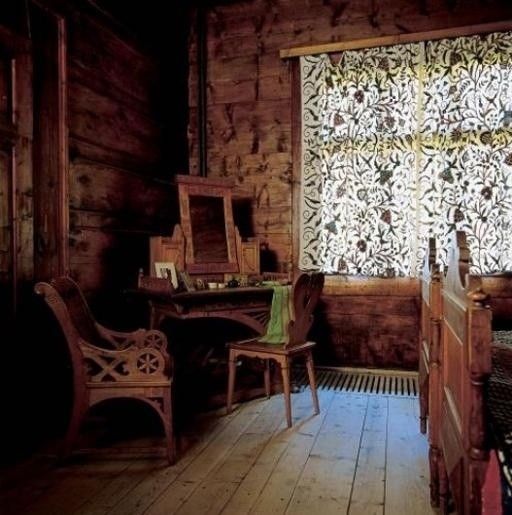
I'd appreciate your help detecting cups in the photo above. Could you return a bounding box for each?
[207,282,225,289]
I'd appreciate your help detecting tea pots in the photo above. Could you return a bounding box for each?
[227,277,241,288]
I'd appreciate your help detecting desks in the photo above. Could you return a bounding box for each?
[140,277,291,401]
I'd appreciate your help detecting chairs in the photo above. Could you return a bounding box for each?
[224,271,327,429]
[36,276,179,468]
